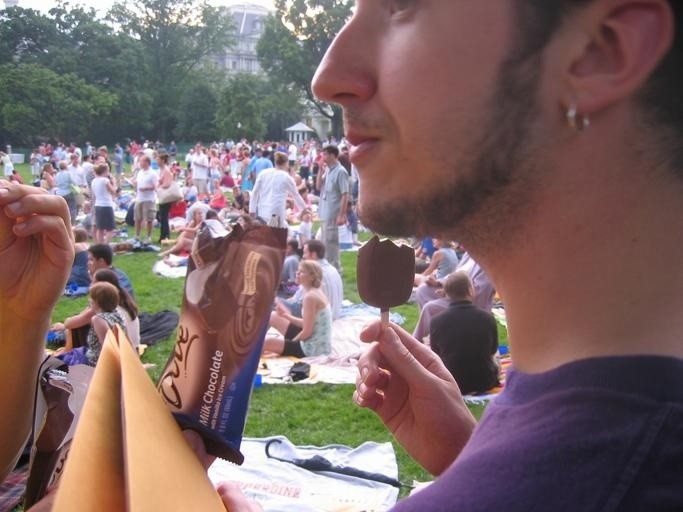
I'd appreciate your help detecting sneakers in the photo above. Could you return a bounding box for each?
[131,235,138,241]
[143,236,151,244]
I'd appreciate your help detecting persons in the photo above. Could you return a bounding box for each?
[82,141,112,172]
[412,252,496,346]
[184,138,250,211]
[218,0,682,512]
[159,202,269,268]
[86,155,114,243]
[261,259,332,358]
[64,269,140,357]
[297,209,312,257]
[98,145,111,172]
[67,229,92,287]
[316,145,349,278]
[87,244,134,302]
[39,154,82,225]
[247,135,288,180]
[287,142,297,173]
[271,240,343,323]
[280,240,299,281]
[249,152,306,230]
[10,170,23,184]
[0,182,218,512]
[55,283,134,367]
[430,273,500,396]
[126,269,127,270]
[31,143,81,178]
[287,167,317,212]
[298,140,322,189]
[0,151,14,181]
[134,155,156,245]
[128,160,182,178]
[416,236,436,259]
[126,138,177,161]
[321,135,354,177]
[114,143,123,174]
[71,204,92,232]
[91,164,116,245]
[154,154,173,246]
[414,237,459,286]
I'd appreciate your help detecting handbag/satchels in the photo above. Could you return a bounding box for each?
[70,184,81,195]
[154,182,184,204]
